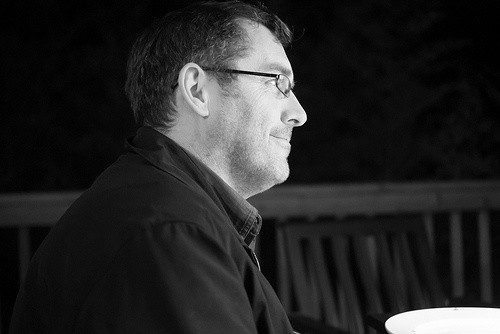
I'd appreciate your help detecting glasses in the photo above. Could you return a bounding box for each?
[171,66,295,98]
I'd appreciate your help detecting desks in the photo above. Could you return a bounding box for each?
[384,307,500,334]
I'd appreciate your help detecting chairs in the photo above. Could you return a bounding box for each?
[277,220,438,334]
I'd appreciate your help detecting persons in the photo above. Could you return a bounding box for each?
[9,0,308,334]
